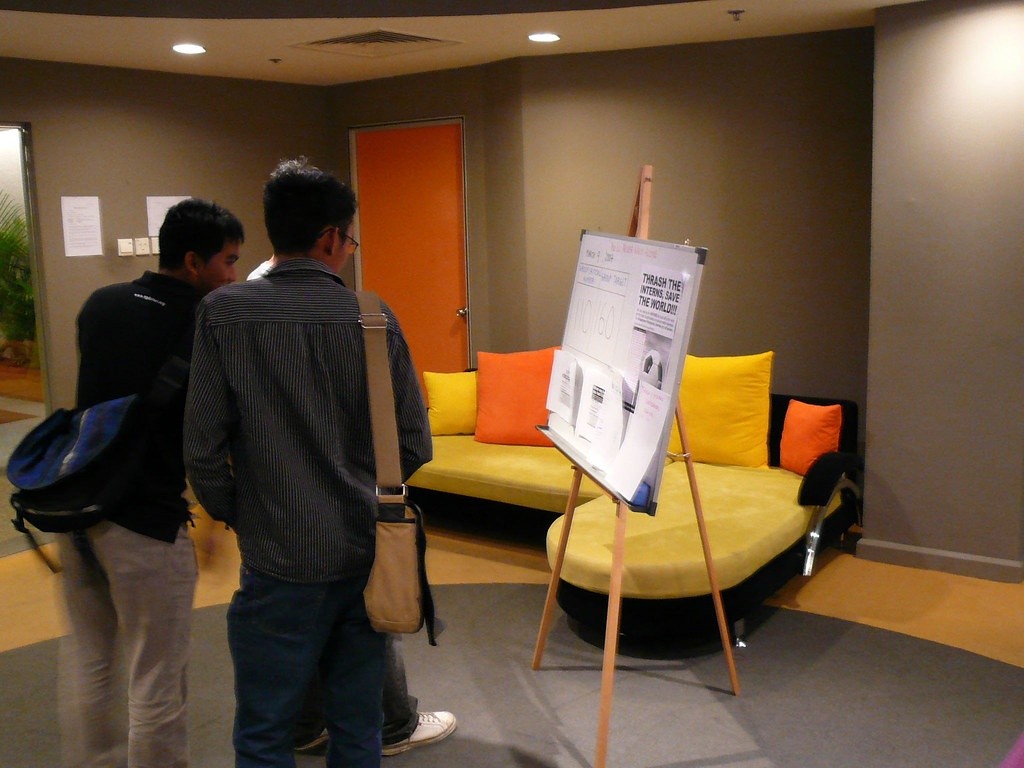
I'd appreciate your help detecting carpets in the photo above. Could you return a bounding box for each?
[0,581,1024,768]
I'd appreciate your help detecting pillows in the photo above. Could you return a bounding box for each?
[779,399,843,476]
[422,370,479,434]
[474,345,563,448]
[665,352,774,469]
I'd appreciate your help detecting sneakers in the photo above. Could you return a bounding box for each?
[381,711,456,757]
[293,729,329,752]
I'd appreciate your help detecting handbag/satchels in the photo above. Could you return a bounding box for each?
[5,391,148,532]
[363,486,436,647]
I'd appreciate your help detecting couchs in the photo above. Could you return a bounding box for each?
[406,367,863,652]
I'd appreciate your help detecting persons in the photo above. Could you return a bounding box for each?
[55,197,245,768]
[183,162,433,768]
[247,253,457,756]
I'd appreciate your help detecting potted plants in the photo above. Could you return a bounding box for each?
[0,189,39,366]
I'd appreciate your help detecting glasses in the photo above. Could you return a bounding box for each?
[319,228,360,255]
